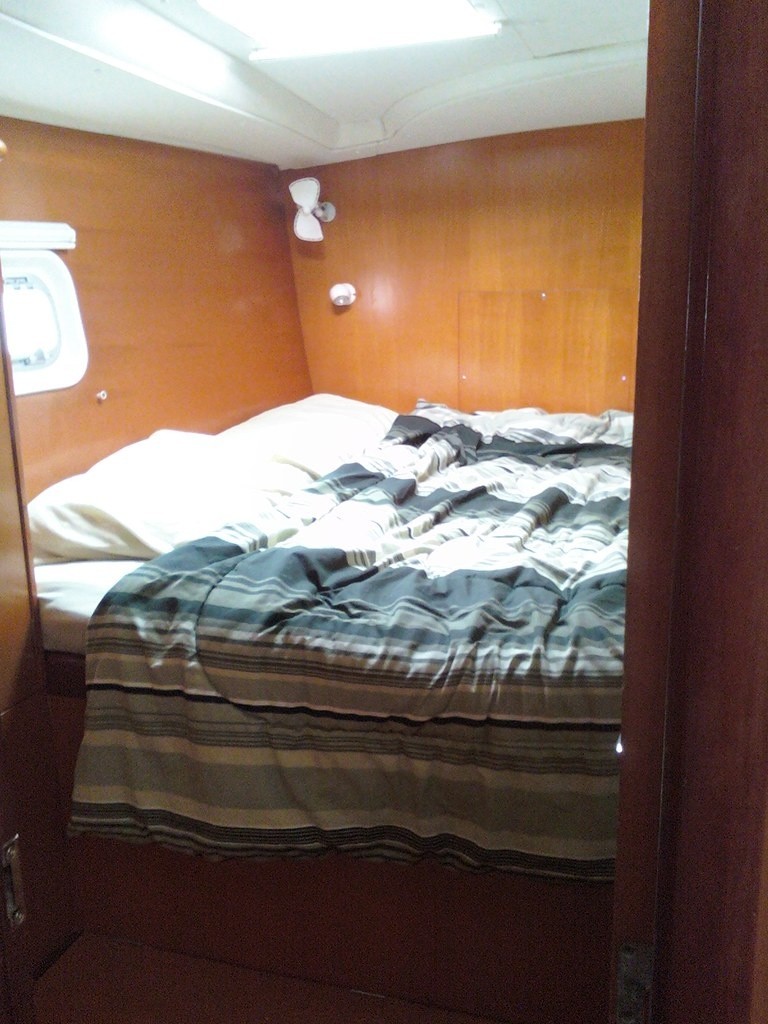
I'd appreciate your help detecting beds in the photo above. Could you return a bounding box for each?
[20,390,634,887]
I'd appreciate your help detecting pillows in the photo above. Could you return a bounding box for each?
[27,428,316,559]
[215,390,402,480]
